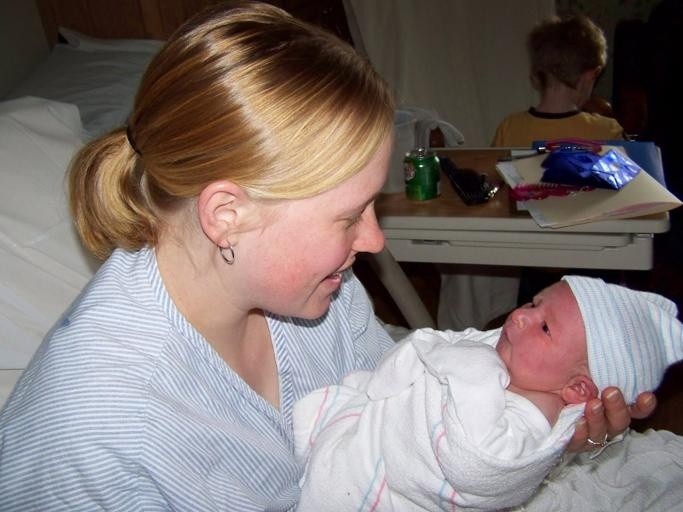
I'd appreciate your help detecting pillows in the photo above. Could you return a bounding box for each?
[5,28,166,140]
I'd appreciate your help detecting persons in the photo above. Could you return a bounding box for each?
[2,1,659,512]
[287,275,681,512]
[490,14,622,145]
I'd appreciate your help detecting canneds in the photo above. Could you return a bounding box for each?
[403,144,441,201]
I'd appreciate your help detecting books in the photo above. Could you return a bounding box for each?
[491,141,682,231]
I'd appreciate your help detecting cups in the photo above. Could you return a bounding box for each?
[378,107,419,196]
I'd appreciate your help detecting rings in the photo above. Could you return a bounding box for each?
[584,434,608,444]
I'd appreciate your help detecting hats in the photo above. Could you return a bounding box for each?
[561,274,683,407]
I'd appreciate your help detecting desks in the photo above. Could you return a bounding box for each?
[524,270,525,277]
[371,147,671,277]
